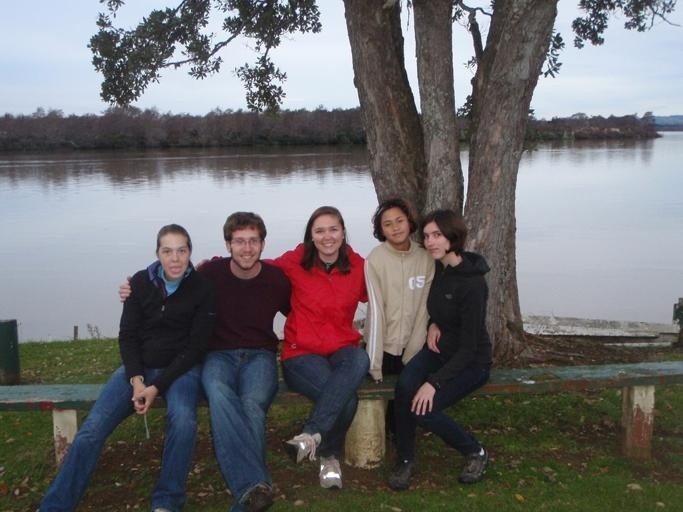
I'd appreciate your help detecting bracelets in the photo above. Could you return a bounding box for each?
[129,374,143,387]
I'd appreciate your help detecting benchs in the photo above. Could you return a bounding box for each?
[0,358,683,471]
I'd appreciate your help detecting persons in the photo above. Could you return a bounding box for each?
[30,222,216,512]
[361,197,435,445]
[386,209,492,493]
[115,210,292,512]
[192,206,368,490]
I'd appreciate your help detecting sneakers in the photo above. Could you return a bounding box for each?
[281,432,322,465]
[458,445,489,483]
[387,457,418,491]
[319,454,343,490]
[240,483,274,512]
